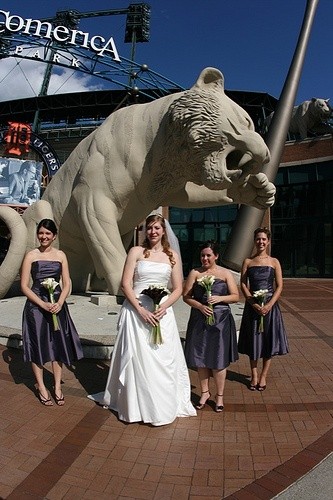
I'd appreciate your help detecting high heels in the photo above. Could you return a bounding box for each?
[37,387,53,406]
[215,393,224,412]
[54,388,65,406]
[196,391,211,410]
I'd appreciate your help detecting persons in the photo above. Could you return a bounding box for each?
[20,219,84,406]
[238,228,290,391]
[86,213,198,426]
[10,161,35,203]
[183,240,239,411]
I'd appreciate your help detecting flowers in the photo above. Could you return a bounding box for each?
[40,278,61,331]
[197,275,216,326]
[249,289,268,333]
[140,285,171,345]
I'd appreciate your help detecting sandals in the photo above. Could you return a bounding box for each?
[258,384,266,391]
[249,384,257,390]
